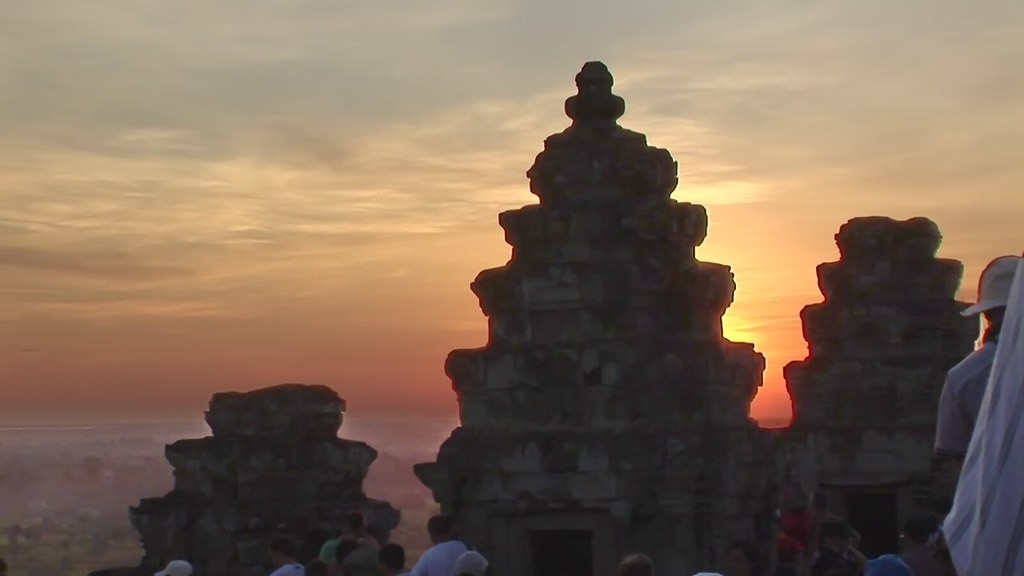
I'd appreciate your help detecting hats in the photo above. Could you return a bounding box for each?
[317,537,344,566]
[451,551,491,576]
[153,560,195,576]
[862,553,915,576]
[958,255,1022,320]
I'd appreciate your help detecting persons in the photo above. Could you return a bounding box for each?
[934,256,1024,576]
[620,484,952,576]
[140,508,487,576]
